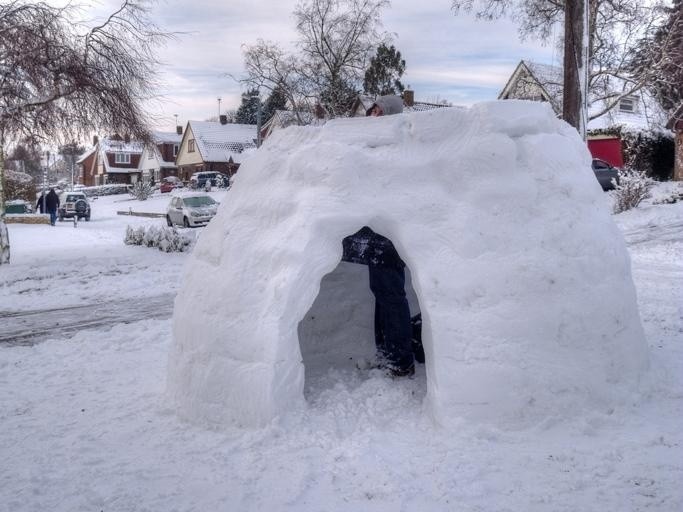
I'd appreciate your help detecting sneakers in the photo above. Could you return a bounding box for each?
[380,348,416,379]
[356,347,393,370]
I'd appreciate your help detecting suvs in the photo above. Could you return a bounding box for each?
[55,191,92,221]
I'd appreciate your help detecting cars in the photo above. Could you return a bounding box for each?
[166,192,221,229]
[159,175,184,194]
[591,157,628,194]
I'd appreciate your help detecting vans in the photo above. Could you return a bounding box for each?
[189,170,230,188]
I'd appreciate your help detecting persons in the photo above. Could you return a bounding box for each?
[46,189,60,225]
[36,192,47,213]
[341,96,425,378]
